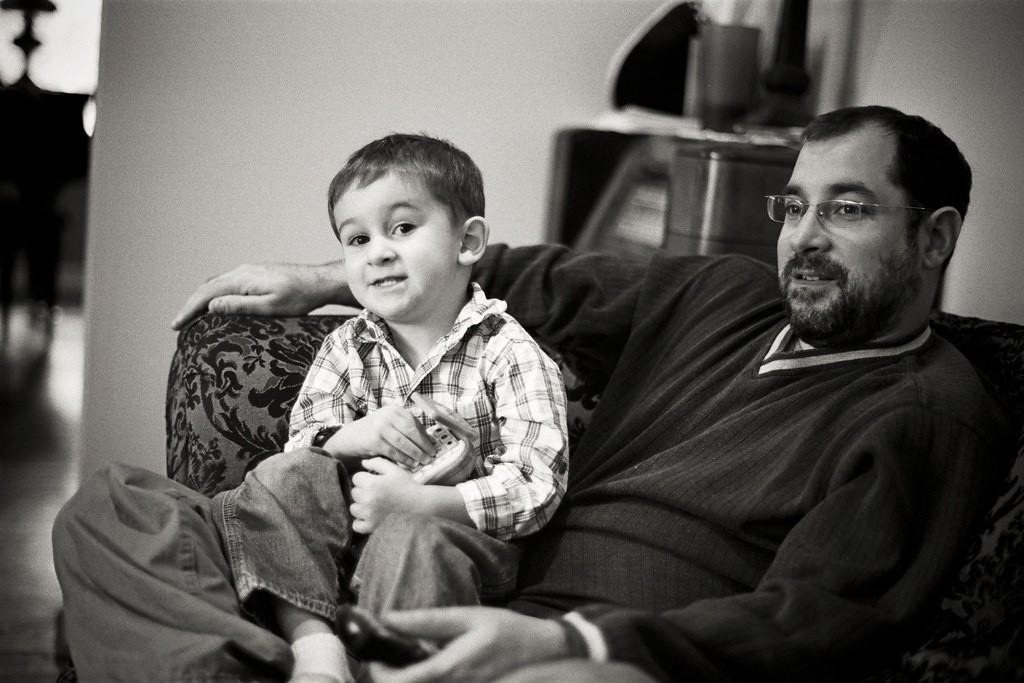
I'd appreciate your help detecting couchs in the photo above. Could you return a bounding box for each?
[169,309,1024,683]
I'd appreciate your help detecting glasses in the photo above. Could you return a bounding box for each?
[764,193,931,229]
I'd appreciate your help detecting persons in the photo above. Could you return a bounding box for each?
[50,105,1001,683]
[209,132,573,683]
